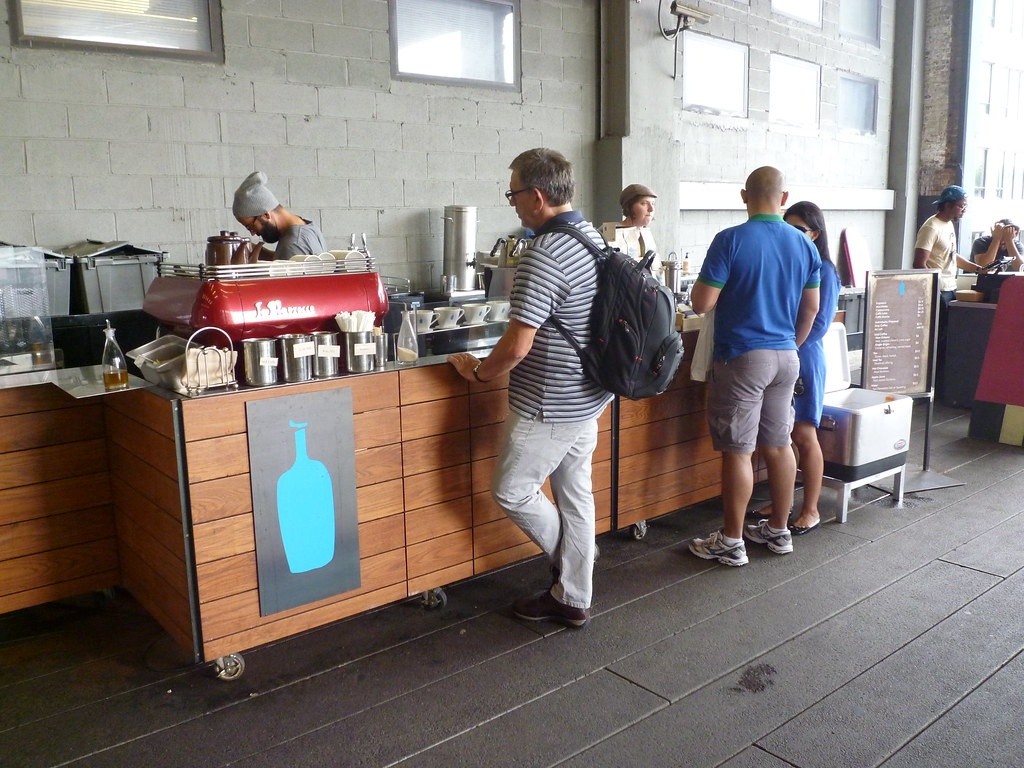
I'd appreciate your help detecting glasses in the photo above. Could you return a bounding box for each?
[954,204,968,212]
[504,187,532,202]
[245,216,257,230]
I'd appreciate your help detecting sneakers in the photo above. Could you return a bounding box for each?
[688,527,749,566]
[744,519,794,554]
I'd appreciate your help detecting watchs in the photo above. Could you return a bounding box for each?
[472,363,491,384]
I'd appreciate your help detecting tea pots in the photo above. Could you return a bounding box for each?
[491,235,533,268]
[205,230,264,265]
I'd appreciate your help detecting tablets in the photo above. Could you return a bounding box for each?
[975,256,1017,274]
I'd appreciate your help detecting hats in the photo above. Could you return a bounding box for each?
[619,183,658,208]
[232,170,280,220]
[930,185,968,209]
[995,220,1019,238]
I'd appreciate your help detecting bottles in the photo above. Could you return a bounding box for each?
[682,253,690,272]
[102,321,129,391]
[28,308,51,365]
[396,310,419,366]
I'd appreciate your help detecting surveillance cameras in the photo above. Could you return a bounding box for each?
[671,0,713,25]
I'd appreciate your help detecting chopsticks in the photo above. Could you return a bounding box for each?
[335,309,376,332]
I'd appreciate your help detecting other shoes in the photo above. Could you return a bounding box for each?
[747,509,794,520]
[547,543,600,589]
[513,589,591,629]
[789,517,821,535]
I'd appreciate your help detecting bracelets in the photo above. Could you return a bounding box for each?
[975,265,981,275]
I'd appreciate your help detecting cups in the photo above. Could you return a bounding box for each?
[434,307,465,326]
[410,309,440,330]
[461,303,491,322]
[240,330,389,386]
[486,301,511,319]
[262,249,366,276]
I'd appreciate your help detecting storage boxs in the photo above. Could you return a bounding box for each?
[0,244,75,317]
[955,290,984,302]
[125,335,202,391]
[54,238,171,314]
[798,322,915,482]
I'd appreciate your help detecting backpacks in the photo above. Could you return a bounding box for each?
[529,224,684,400]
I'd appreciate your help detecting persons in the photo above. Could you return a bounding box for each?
[913,185,990,395]
[231,172,327,261]
[747,201,840,538]
[971,218,1024,303]
[619,184,665,289]
[448,149,613,625]
[689,165,820,568]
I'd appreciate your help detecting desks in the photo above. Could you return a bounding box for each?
[940,299,997,426]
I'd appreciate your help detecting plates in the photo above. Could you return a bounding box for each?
[419,318,510,332]
